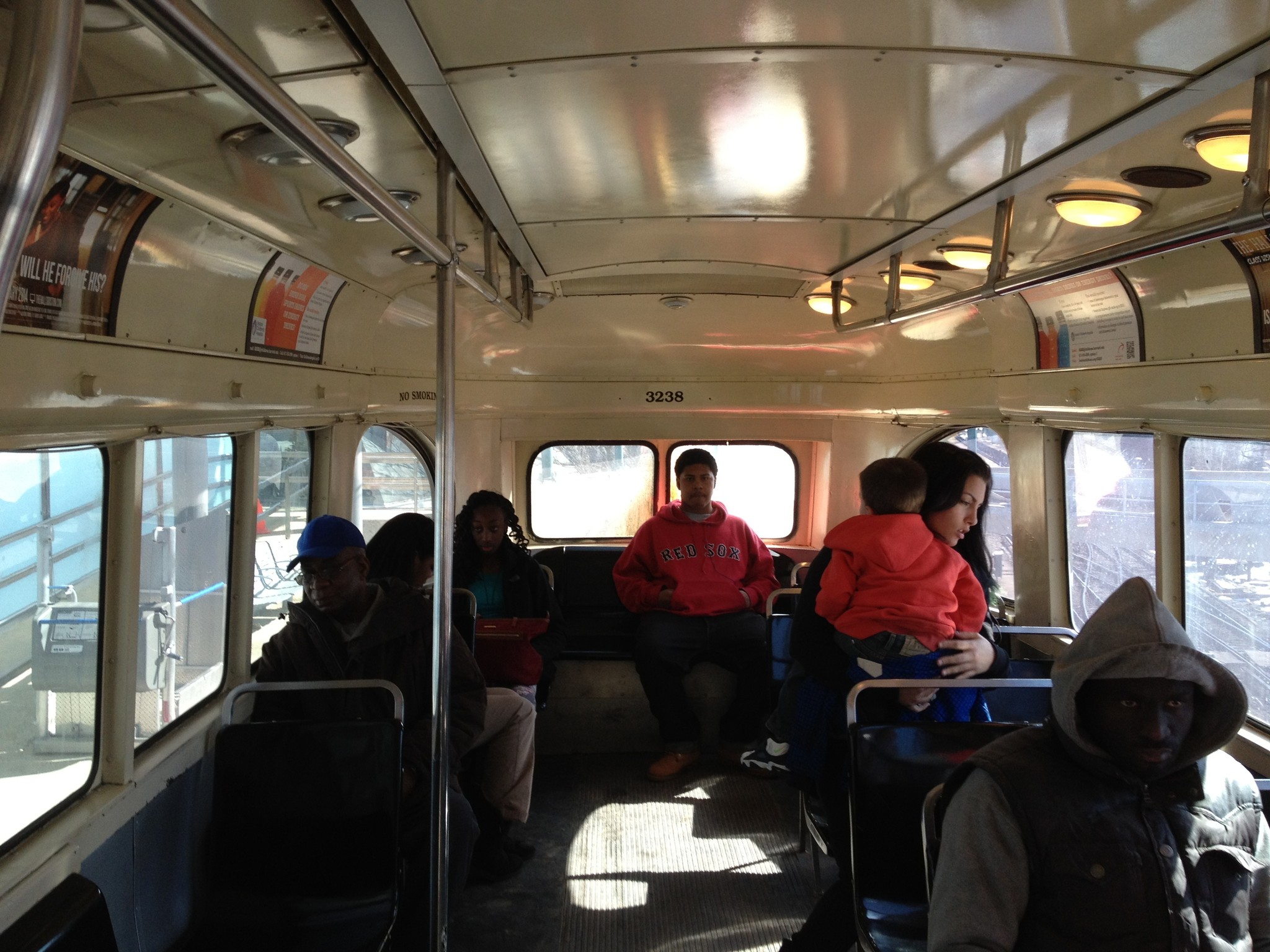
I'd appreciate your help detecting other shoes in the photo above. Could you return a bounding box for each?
[468,844,524,885]
[482,827,534,862]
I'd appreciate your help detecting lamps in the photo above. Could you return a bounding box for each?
[1181,125,1254,173]
[80,0,144,35]
[219,116,361,166]
[936,245,1016,270]
[392,242,469,266]
[659,295,693,310]
[504,292,555,313]
[878,270,940,291]
[318,190,421,224]
[1045,192,1155,229]
[803,293,856,315]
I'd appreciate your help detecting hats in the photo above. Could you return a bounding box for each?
[286,514,366,572]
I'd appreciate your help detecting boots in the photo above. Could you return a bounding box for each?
[645,741,700,782]
[719,741,773,777]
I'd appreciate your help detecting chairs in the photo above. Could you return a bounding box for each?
[173,679,409,952]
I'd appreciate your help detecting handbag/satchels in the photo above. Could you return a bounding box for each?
[473,613,550,685]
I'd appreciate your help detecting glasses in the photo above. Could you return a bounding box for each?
[294,555,360,586]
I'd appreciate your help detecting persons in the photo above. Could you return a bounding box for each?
[19,174,79,328]
[360,512,536,864]
[611,446,781,781]
[924,575,1270,952]
[775,440,1013,952]
[451,491,557,731]
[254,514,491,940]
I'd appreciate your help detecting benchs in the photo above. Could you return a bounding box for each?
[533,547,800,663]
[767,561,1270,952]
